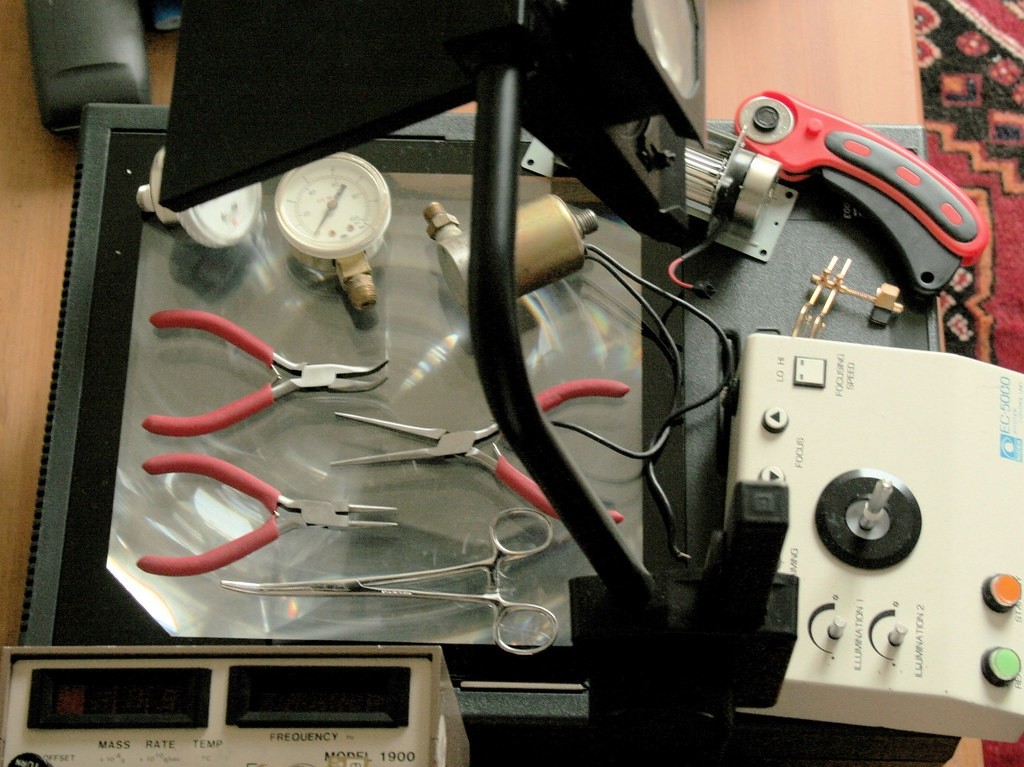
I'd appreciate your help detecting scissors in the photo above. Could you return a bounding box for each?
[220,508,557,654]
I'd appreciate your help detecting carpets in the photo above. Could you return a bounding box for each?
[910,0,1024,767]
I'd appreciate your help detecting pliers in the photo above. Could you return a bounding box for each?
[142,308,390,438]
[137,454,399,577]
[330,378,630,524]
